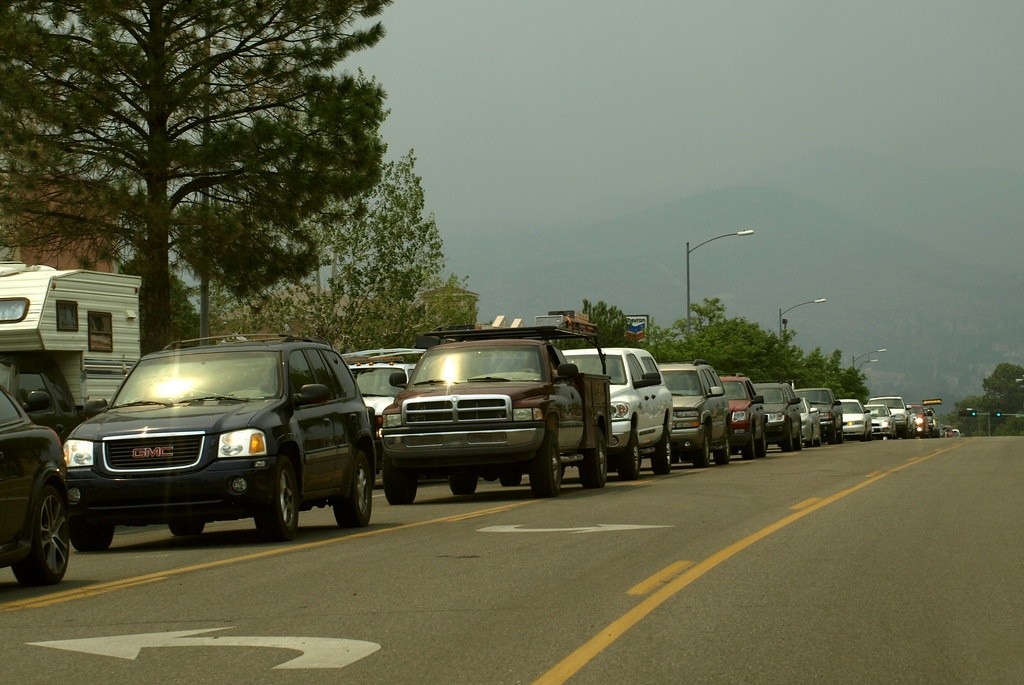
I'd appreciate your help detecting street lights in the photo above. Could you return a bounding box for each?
[852,348,887,369]
[687,229,754,336]
[782,318,788,330]
[779,297,827,340]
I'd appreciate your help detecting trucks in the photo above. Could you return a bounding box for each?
[0,259,143,445]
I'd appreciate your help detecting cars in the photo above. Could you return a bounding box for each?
[800,399,823,447]
[925,407,960,438]
[910,404,930,436]
[909,410,917,437]
[836,399,872,441]
[1,383,69,590]
[864,405,896,439]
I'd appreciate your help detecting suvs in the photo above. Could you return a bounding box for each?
[61,332,373,549]
[751,382,803,452]
[559,350,674,477]
[716,372,769,460]
[794,388,844,446]
[868,397,913,438]
[660,360,732,468]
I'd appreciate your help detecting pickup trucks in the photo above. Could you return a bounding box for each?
[379,308,613,506]
[343,348,427,471]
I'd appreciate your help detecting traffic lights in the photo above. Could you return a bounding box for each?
[991,412,1001,417]
[959,410,977,416]
